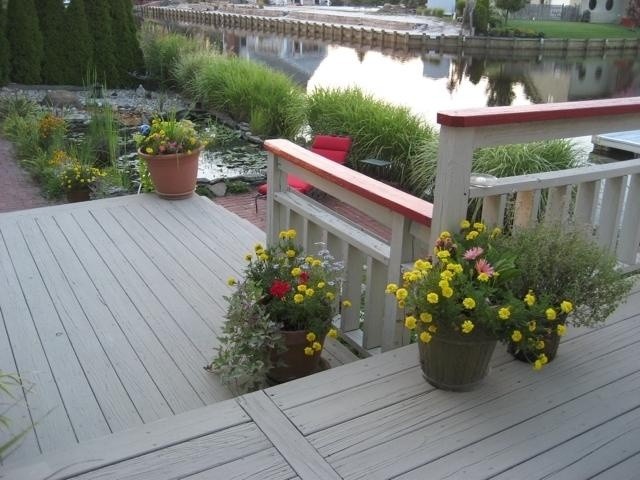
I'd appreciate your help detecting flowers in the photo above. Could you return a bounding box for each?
[56,164,110,192]
[130,82,217,170]
[382,219,573,370]
[202,227,352,399]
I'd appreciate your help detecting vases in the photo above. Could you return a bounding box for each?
[66,184,92,202]
[138,145,206,200]
[415,315,499,393]
[262,318,331,387]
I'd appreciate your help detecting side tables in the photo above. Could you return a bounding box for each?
[357,157,395,187]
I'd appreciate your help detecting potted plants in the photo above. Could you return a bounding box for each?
[497,220,640,366]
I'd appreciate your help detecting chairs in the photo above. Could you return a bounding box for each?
[254,133,353,214]
